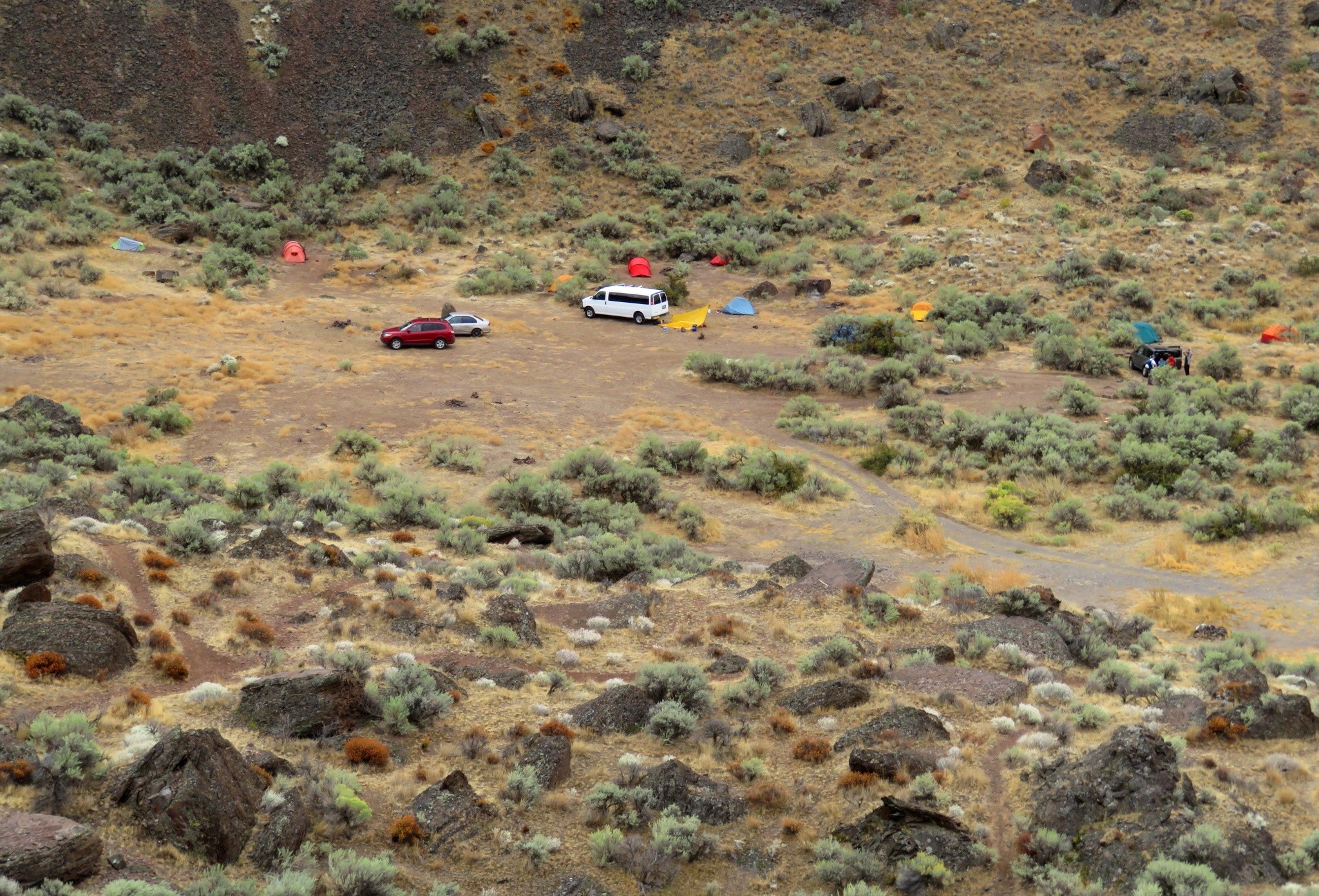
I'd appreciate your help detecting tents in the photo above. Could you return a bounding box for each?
[1262,325,1299,344]
[1130,322,1162,344]
[628,257,654,278]
[549,273,573,294]
[909,302,934,321]
[721,297,757,316]
[283,241,307,264]
[710,254,733,265]
[110,237,147,252]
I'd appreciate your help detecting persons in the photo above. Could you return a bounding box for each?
[1166,354,1177,369]
[1158,358,1167,367]
[1183,348,1193,376]
[1148,355,1158,385]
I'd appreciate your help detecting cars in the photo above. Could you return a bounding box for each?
[439,313,491,339]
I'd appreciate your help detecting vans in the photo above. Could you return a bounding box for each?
[580,283,669,325]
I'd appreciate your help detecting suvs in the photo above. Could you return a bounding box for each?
[381,318,456,350]
[1129,344,1183,377]
